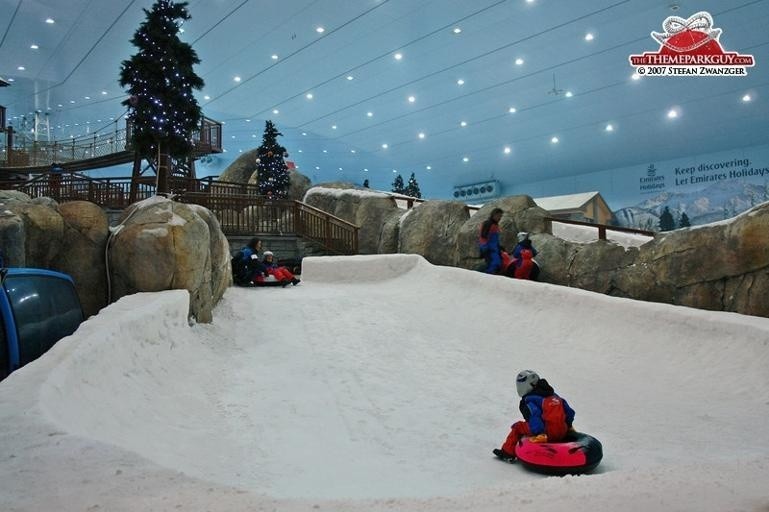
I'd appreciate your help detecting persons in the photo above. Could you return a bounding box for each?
[232,237,269,287]
[492,368,576,463]
[478,207,512,274]
[259,249,302,288]
[506,230,541,281]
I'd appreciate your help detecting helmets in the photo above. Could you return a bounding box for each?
[515,369,540,396]
[263,250,274,259]
[516,231,529,243]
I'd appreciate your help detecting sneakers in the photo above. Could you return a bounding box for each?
[281,279,302,288]
[492,447,516,463]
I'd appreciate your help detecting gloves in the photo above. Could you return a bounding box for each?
[528,434,548,443]
[570,426,577,433]
